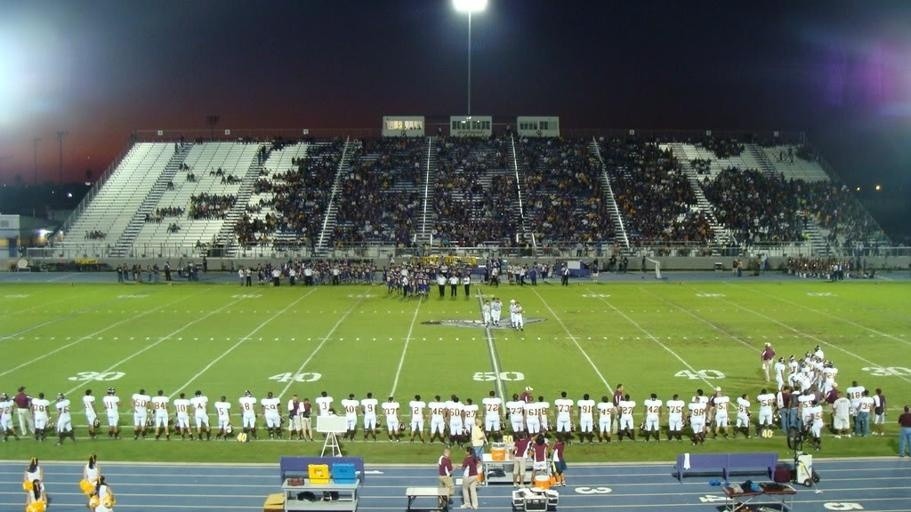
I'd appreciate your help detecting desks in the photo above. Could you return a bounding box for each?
[723,484,797,512]
[282,477,359,512]
[405,486,452,512]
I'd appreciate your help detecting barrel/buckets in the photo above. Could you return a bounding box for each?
[535,475,550,489]
[491,446,506,462]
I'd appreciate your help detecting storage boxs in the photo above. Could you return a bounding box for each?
[263,493,285,512]
[309,464,358,483]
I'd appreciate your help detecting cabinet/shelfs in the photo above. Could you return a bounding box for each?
[483,453,549,486]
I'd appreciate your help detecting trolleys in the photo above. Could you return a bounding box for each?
[792,431,820,487]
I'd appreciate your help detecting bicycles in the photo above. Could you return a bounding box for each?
[788,412,821,450]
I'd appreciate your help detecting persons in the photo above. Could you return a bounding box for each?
[82,454,114,512]
[435,418,567,509]
[0,381,886,444]
[1,131,911,299]
[482,297,523,331]
[897,405,911,458]
[761,342,839,403]
[23,457,48,512]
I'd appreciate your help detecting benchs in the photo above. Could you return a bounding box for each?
[281,457,365,485]
[671,451,778,481]
[48,143,892,261]
